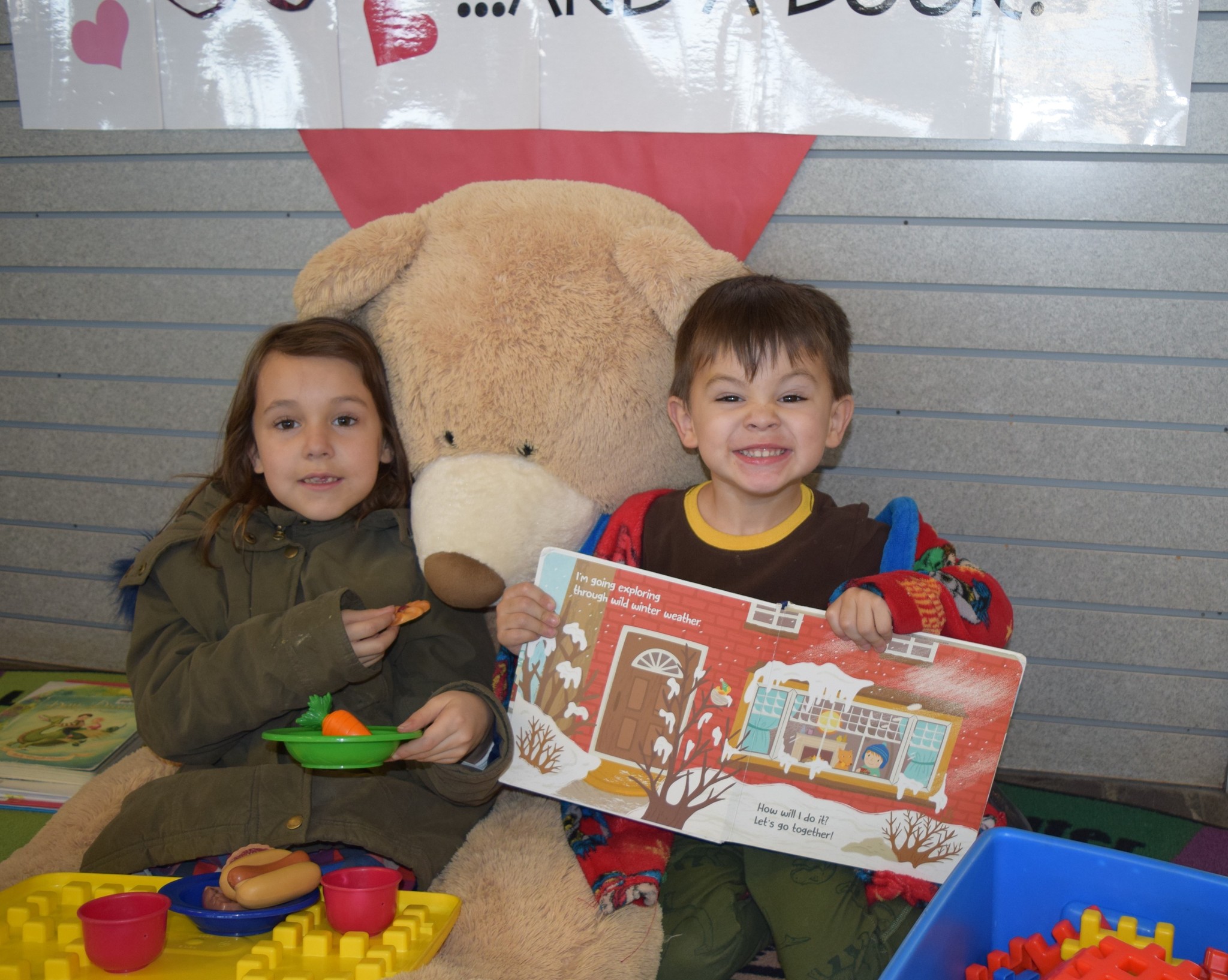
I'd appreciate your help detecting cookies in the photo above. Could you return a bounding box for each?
[390,600,431,625]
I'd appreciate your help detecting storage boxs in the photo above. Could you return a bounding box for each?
[879,826,1228,980]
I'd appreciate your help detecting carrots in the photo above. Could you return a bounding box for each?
[296,692,372,736]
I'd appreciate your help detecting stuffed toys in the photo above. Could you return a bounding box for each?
[0,177,747,979]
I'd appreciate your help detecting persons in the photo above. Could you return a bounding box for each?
[83,318,514,892]
[494,276,1013,979]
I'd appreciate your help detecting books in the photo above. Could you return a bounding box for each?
[0,678,144,812]
[497,547,1028,886]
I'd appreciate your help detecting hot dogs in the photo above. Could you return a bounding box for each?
[219,849,322,909]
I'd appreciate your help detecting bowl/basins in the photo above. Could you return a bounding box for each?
[77,891,171,973]
[321,866,403,937]
[157,872,321,937]
[262,725,423,769]
